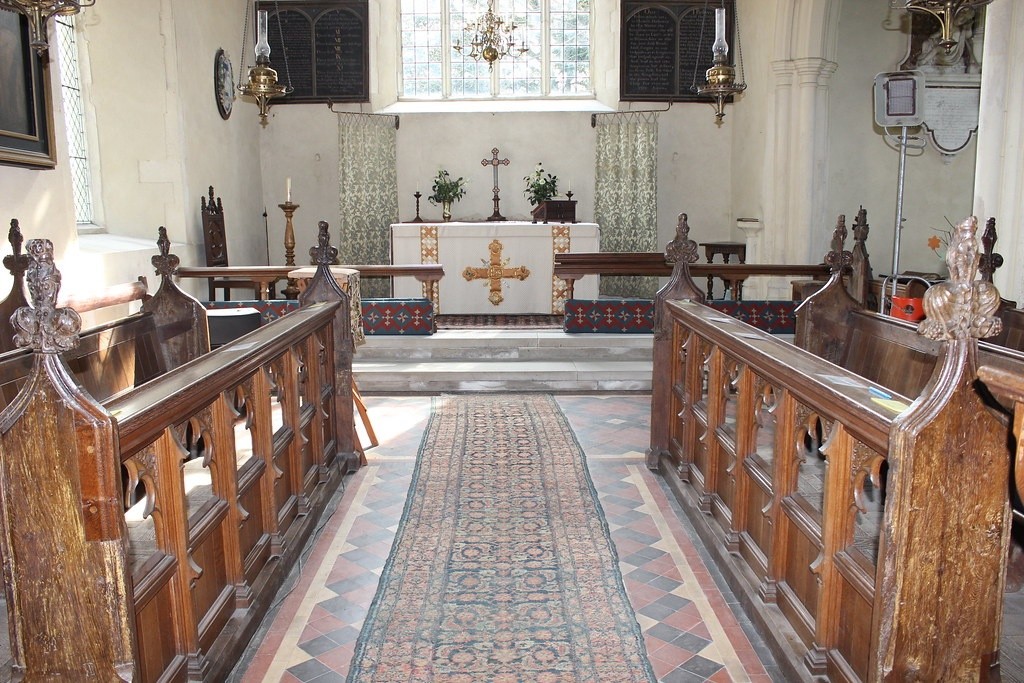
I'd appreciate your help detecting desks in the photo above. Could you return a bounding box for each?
[699,241,746,300]
[389,221,601,314]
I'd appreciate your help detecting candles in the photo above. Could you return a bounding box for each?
[287,178,291,201]
[569,180,570,191]
[417,183,418,192]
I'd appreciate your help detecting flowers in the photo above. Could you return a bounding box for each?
[523,163,563,206]
[428,170,471,206]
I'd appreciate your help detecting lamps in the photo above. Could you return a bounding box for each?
[453,0,529,72]
[237,0,294,121]
[690,0,747,120]
[888,0,994,55]
[0,0,95,57]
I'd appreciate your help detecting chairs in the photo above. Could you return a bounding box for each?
[201,185,280,301]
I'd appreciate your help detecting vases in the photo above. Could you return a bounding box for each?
[443,200,451,222]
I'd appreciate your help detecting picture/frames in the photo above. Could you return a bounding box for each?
[0,10,59,169]
